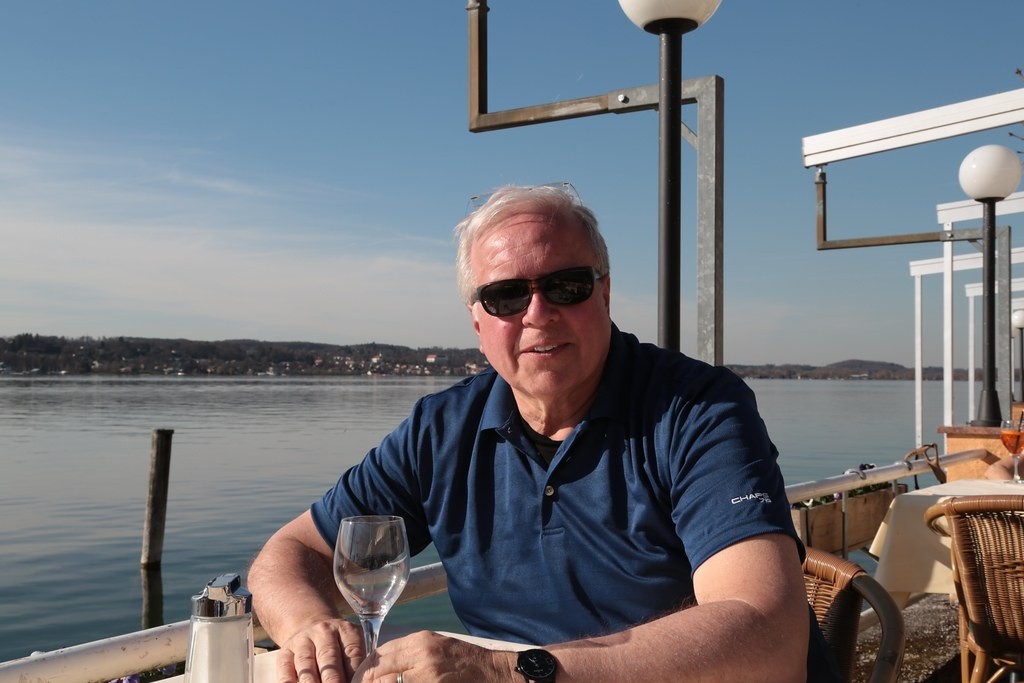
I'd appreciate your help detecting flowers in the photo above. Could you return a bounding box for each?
[104,660,185,683]
[791,463,893,511]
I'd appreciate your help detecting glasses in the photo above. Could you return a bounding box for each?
[471,266,603,317]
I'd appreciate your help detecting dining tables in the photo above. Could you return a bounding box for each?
[868,479,1024,611]
[145,616,544,683]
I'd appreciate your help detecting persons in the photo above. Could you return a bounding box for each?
[246,181,809,683]
[985,449,1024,480]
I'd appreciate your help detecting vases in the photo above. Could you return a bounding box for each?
[790,484,908,556]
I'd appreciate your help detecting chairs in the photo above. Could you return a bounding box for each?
[924,495,1024,683]
[801,546,906,683]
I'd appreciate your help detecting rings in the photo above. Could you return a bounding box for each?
[397,672,403,683]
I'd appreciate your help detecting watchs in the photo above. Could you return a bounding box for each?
[514,649,557,683]
